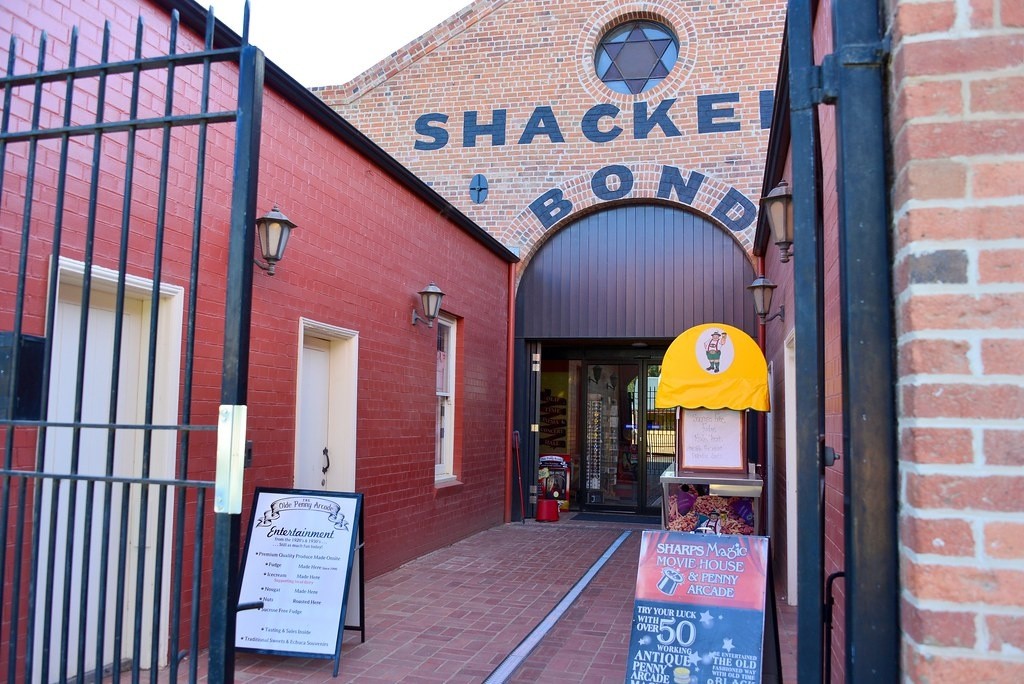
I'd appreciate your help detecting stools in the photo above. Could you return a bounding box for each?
[535,498,559,521]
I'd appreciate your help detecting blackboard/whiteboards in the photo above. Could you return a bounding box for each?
[678,407,747,474]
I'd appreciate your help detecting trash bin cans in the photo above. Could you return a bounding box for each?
[536,499,559,521]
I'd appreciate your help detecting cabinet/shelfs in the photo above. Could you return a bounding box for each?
[660,463,764,536]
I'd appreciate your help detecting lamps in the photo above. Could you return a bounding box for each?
[253,205,298,276]
[413,281,446,328]
[746,275,785,324]
[760,180,794,263]
